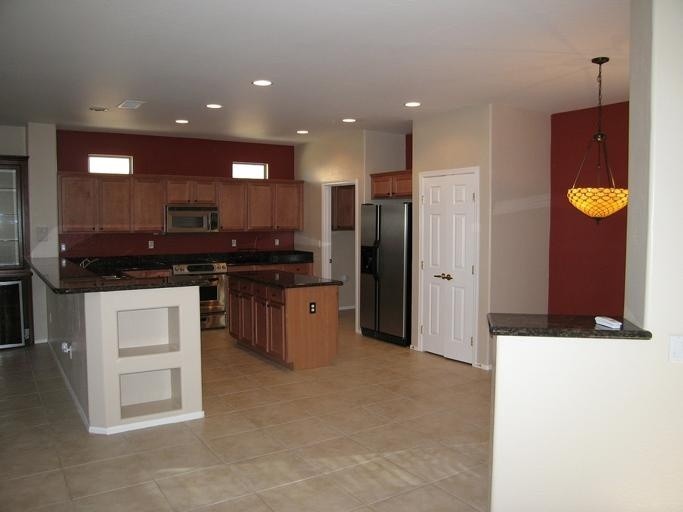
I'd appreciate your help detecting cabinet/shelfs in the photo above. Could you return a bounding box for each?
[218,178,305,232]
[227,277,339,372]
[332,186,355,231]
[369,169,412,200]
[57,173,165,234]
[165,176,218,210]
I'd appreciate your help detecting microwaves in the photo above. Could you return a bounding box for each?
[167,204,220,233]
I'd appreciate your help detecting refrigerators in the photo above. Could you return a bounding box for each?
[360,201,411,347]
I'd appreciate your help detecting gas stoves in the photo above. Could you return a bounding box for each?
[161,259,228,276]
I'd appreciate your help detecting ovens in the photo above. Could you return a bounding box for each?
[169,275,226,329]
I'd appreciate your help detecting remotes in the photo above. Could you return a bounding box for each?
[595,315,623,329]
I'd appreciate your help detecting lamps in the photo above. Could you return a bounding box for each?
[566,56,628,223]
